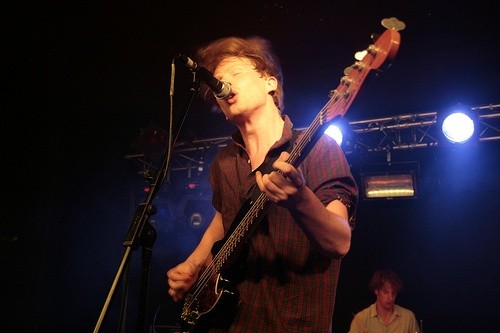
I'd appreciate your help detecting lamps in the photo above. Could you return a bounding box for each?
[325,124,344,147]
[436,103,481,150]
[360,145,421,202]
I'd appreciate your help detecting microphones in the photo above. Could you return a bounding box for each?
[176,54,232,99]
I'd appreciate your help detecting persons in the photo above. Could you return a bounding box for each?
[348,269,420,333]
[168,37,359,333]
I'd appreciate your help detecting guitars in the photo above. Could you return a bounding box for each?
[152,28,401,333]
